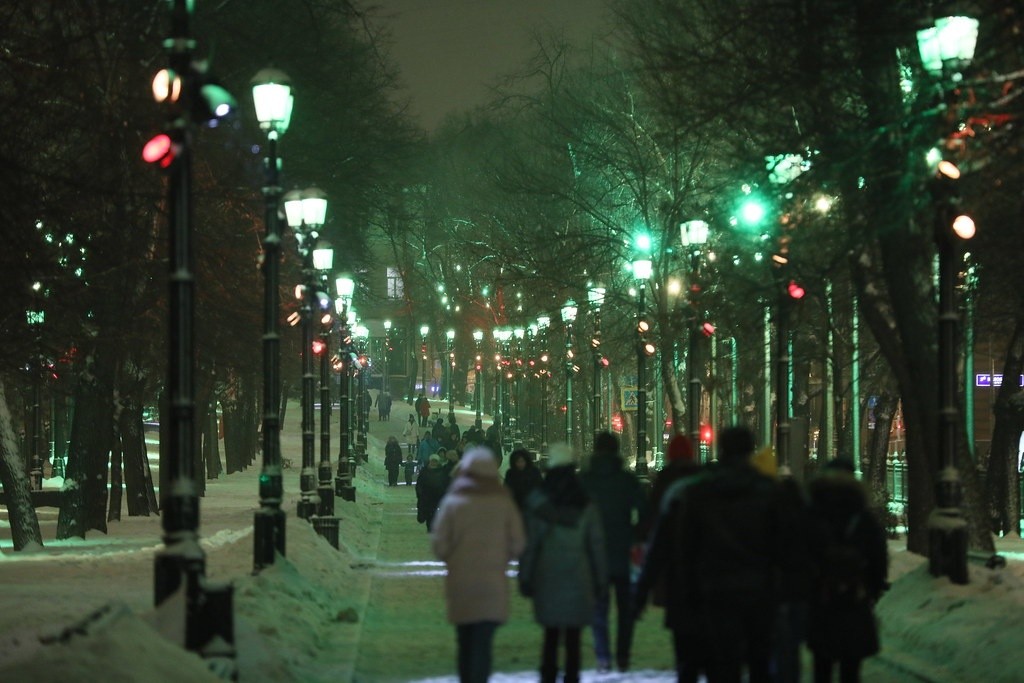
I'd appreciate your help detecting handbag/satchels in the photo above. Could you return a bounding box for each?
[407,430,411,435]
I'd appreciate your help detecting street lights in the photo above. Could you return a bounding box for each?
[246,72,373,584]
[378,17,988,584]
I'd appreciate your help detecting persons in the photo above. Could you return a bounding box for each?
[372,388,888,683]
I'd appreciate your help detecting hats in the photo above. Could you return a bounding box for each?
[409,414,414,417]
[429,454,440,460]
[667,435,693,458]
[464,448,498,474]
[754,444,779,478]
[549,445,572,464]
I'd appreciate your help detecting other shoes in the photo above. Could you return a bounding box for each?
[597,661,629,675]
